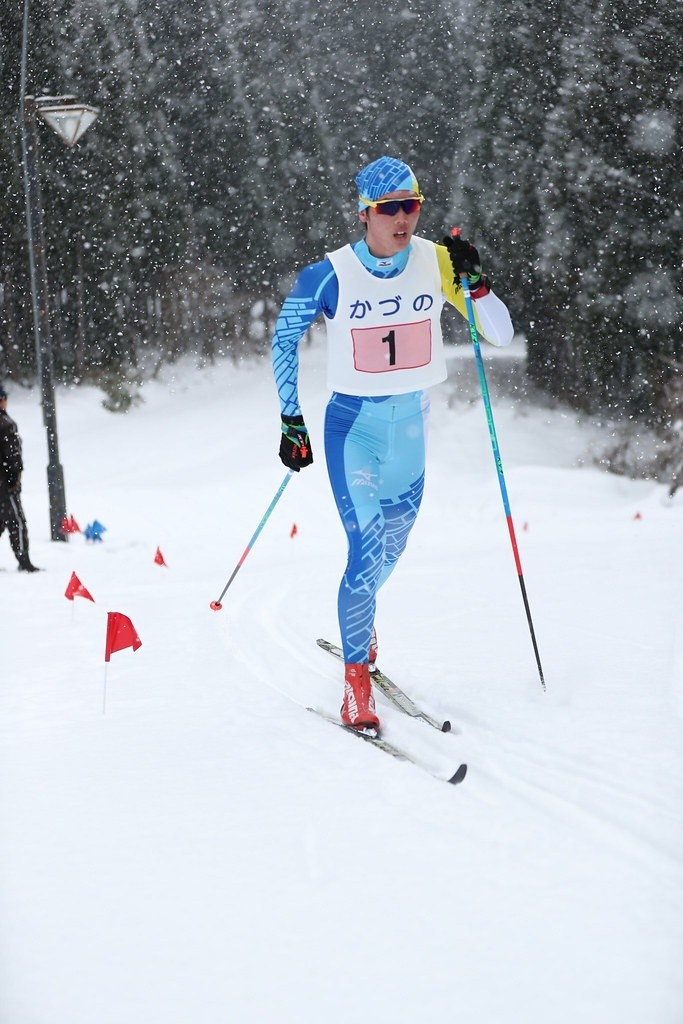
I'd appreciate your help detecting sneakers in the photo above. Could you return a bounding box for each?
[343,663,379,730]
[367,627,378,663]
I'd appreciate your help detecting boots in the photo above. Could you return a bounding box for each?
[18,553,39,573]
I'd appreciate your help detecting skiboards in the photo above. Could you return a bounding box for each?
[304,638,468,785]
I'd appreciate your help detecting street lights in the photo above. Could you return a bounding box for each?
[20,90,96,542]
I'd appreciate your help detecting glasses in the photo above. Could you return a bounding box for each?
[376,200,421,216]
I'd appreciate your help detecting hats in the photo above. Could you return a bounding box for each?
[353,156,420,213]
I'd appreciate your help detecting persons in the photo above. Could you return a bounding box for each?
[0,386,43,573]
[271,157,513,736]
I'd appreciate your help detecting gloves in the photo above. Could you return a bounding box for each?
[279,414,313,473]
[443,236,483,290]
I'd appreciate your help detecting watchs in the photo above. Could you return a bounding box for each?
[470,275,491,302]
